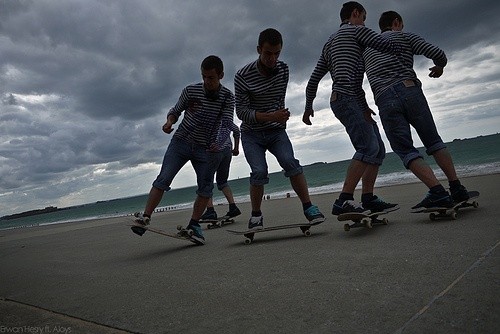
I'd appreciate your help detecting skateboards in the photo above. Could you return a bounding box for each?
[198,218,234,228]
[410,191,480,219]
[337,211,389,231]
[225,217,327,244]
[121,211,200,243]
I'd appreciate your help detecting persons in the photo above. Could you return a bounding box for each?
[234,28,326,228]
[302,0,402,215]
[360,10,469,213]
[131,54,235,243]
[199,120,240,219]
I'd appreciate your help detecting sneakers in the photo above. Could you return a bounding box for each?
[248,216,263,232]
[186,224,207,245]
[409,191,454,213]
[449,185,480,203]
[202,210,217,219]
[362,195,401,216]
[304,205,327,224]
[131,217,151,236]
[225,206,241,217]
[332,199,371,215]
